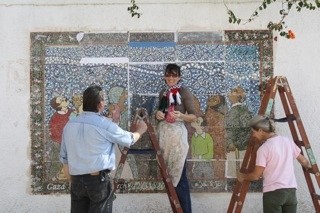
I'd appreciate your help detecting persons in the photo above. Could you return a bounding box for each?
[237,116,317,213]
[155,64,198,213]
[61,85,148,213]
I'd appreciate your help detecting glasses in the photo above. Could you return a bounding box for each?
[165,73,179,77]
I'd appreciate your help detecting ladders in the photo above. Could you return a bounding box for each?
[113,107,183,213]
[227,76,320,213]
